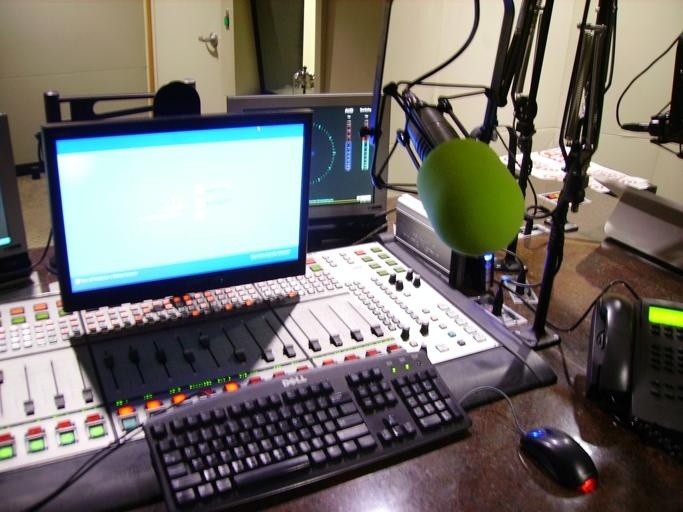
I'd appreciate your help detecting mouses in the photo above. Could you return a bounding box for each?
[520,426,601,494]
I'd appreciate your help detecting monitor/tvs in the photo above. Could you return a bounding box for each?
[227,92,390,221]
[0,114,28,258]
[41,106,314,312]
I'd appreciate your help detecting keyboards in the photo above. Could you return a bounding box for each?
[143,351,473,512]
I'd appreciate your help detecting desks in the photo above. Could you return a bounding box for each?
[0,176,683,511]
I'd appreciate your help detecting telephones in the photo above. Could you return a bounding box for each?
[586,293,683,434]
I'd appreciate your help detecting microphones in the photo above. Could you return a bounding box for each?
[402,90,526,257]
